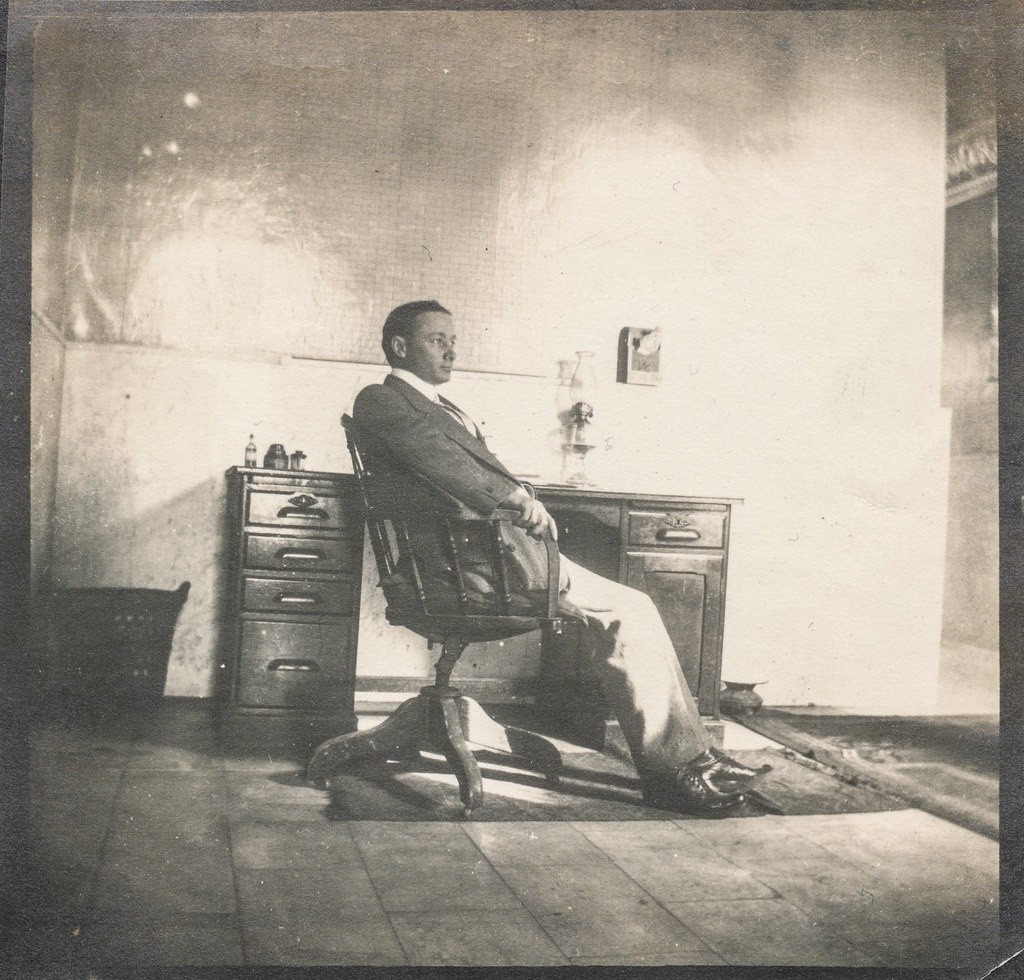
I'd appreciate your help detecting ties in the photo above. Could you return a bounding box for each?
[434,396,477,439]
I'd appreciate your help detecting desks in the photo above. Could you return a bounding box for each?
[212,467,742,755]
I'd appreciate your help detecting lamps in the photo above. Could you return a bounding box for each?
[563,351,602,487]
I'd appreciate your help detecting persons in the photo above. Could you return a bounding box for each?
[351,300,774,818]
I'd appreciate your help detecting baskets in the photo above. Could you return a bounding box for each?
[36,581,191,709]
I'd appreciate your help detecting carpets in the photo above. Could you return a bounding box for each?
[729,712,1000,843]
[324,748,906,821]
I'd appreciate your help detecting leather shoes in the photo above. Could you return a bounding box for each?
[642,763,751,818]
[685,747,773,791]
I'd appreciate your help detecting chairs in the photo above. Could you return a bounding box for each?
[307,414,588,817]
